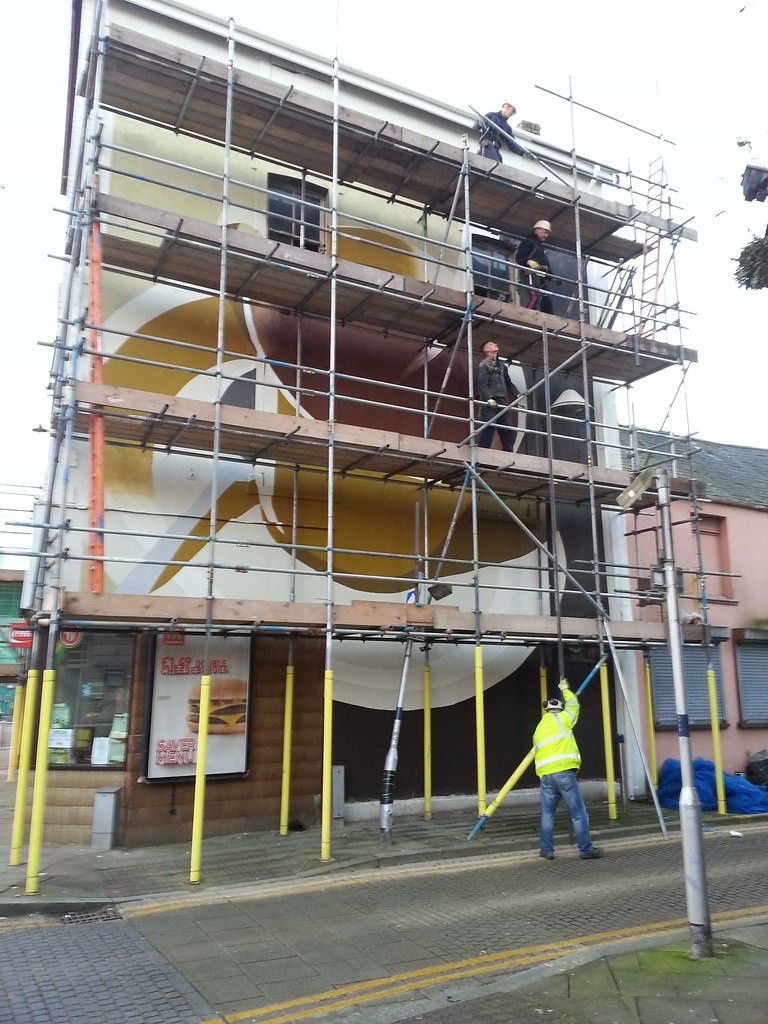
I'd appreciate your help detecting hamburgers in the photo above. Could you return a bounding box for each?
[188,678,247,734]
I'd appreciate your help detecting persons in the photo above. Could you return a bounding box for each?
[532,678,605,860]
[476,341,521,452]
[472,101,536,163]
[515,220,555,313]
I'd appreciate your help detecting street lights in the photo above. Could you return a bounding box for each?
[615,466,712,961]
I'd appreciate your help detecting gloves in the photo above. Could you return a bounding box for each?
[517,390,530,398]
[487,399,498,410]
[558,678,568,691]
[527,260,539,270]
[523,152,535,161]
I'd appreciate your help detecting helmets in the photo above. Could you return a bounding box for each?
[543,698,563,709]
[533,220,551,233]
[502,99,519,114]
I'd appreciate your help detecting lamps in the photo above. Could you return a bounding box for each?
[428,583,453,601]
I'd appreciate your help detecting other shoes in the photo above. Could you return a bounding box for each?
[540,849,554,859]
[580,847,605,858]
